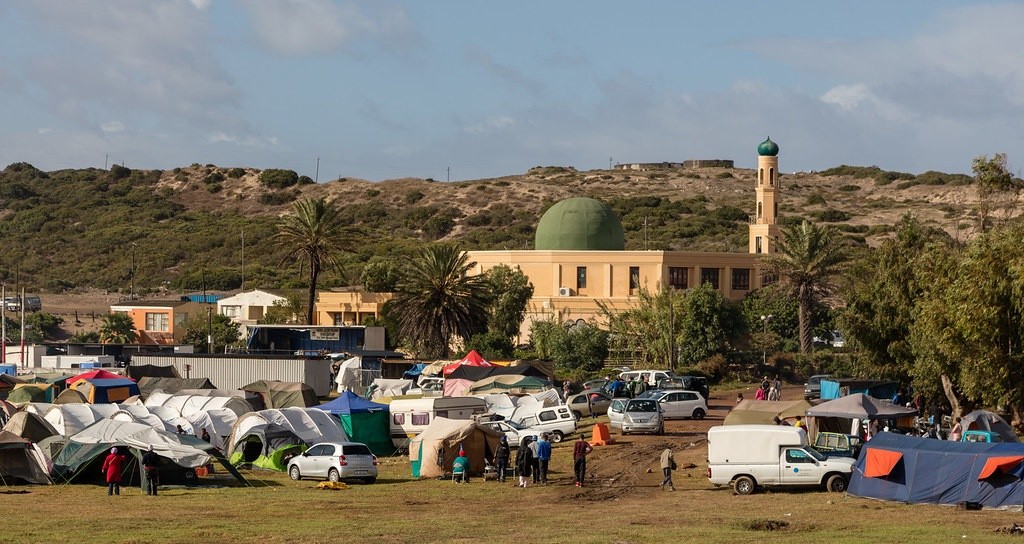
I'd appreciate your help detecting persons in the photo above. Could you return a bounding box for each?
[528,434,541,485]
[537,431,546,443]
[452,451,470,484]
[492,436,510,483]
[562,380,573,400]
[537,434,551,485]
[572,434,593,487]
[604,373,651,400]
[141,444,160,496]
[102,447,126,496]
[658,445,677,492]
[201,428,210,444]
[736,374,782,405]
[515,438,533,488]
[862,384,962,443]
[175,425,186,435]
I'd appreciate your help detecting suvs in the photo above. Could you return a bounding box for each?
[477,414,555,449]
[802,374,834,400]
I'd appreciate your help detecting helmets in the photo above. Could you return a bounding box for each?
[532,435,538,440]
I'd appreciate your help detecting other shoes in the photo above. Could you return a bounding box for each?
[669,487,676,491]
[659,483,665,491]
[576,482,579,486]
[580,484,585,487]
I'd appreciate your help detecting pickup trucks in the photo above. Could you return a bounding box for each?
[516,404,579,444]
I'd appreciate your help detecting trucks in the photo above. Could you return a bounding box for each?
[0,293,42,313]
[790,431,867,459]
[960,429,1005,443]
[388,393,491,450]
[705,424,856,495]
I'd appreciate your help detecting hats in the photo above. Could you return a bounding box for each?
[500,437,505,440]
[460,451,464,457]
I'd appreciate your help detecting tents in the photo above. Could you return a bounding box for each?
[0,350,558,486]
[722,392,920,450]
[948,408,1019,443]
[846,430,1024,510]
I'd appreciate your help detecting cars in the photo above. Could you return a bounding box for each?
[405,376,445,395]
[286,442,378,484]
[639,389,708,420]
[607,397,666,436]
[565,365,710,422]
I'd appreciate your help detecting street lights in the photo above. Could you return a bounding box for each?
[760,314,774,364]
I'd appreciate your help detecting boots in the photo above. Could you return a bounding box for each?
[518,476,524,486]
[524,478,527,487]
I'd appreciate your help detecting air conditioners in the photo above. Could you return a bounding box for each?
[560,288,571,297]
